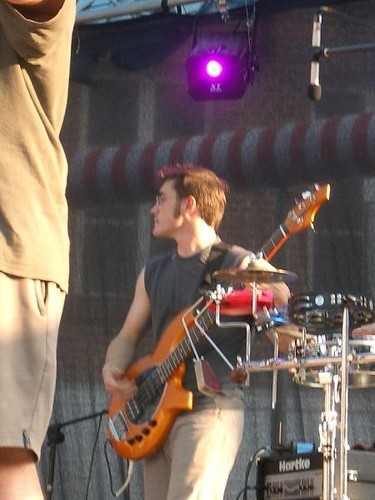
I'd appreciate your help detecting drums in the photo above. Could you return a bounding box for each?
[286,337,375,389]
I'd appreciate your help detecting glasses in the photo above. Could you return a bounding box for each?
[156,192,176,207]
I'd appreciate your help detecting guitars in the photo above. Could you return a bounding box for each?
[104,182,331,461]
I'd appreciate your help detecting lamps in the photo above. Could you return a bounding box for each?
[184,0,257,101]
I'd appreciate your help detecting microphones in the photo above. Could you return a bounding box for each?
[306,9,322,102]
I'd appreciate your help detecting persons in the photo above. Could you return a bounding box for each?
[1,0,75,499]
[100,165,291,500]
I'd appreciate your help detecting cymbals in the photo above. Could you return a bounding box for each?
[287,290,375,331]
[211,257,298,284]
